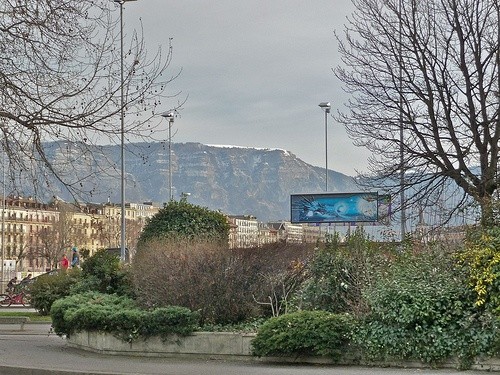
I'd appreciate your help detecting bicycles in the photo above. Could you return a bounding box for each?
[0,283,32,308]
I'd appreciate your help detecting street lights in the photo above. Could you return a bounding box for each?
[115,0,139,258]
[318,101,332,191]
[159,111,174,198]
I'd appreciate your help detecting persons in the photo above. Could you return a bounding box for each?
[8,277,17,293]
[23,274,32,281]
[61,254,68,269]
[71,247,79,269]
